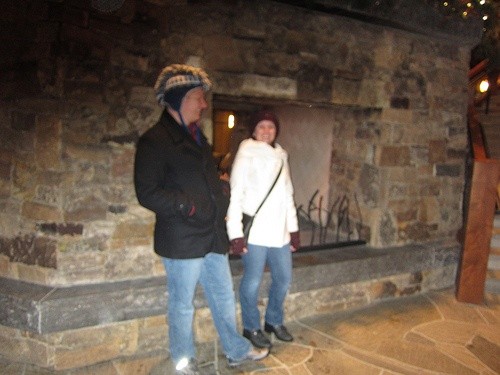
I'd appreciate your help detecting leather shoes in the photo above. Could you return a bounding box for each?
[264,322,293,342]
[242,329,272,349]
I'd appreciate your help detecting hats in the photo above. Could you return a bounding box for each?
[155,65,211,111]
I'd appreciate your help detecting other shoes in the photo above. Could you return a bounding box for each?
[172,358,194,374]
[228,345,269,366]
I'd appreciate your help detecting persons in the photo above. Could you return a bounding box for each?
[134,65,270,375]
[222,110,299,350]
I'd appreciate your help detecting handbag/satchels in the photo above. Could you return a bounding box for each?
[243,213,254,244]
